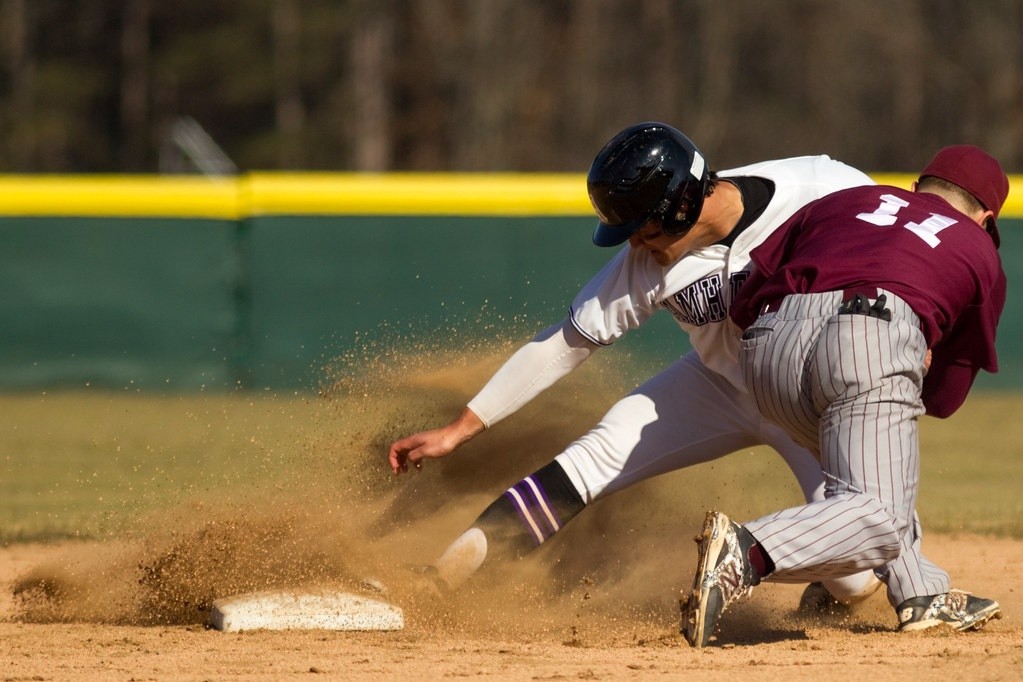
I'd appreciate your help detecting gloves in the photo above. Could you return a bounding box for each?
[845,292,891,321]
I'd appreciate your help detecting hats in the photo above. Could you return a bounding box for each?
[919,144,1010,250]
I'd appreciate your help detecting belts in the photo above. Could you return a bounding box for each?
[760,286,877,315]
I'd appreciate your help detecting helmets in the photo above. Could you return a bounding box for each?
[587,122,709,247]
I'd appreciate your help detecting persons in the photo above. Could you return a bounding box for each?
[681,146,1009,648]
[359,122,887,624]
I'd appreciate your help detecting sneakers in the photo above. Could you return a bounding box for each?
[679,510,756,648]
[895,588,1001,633]
